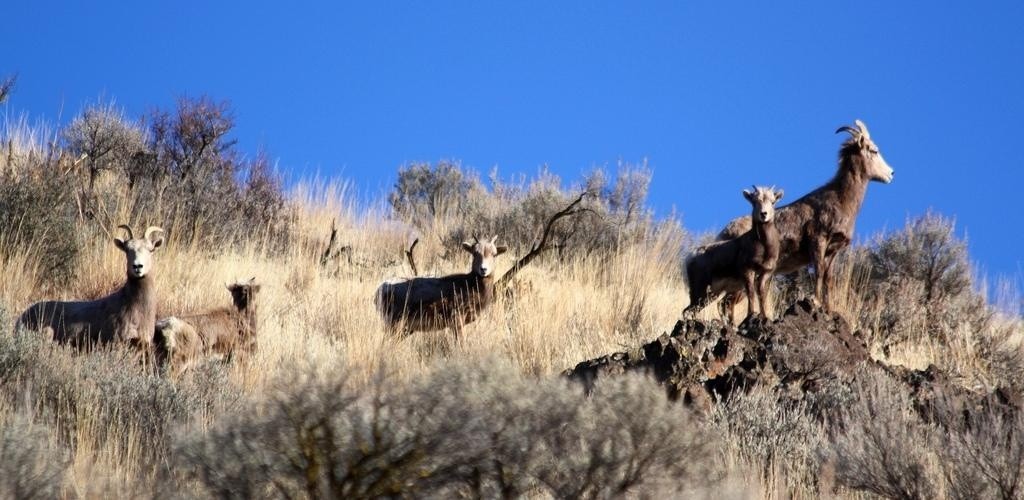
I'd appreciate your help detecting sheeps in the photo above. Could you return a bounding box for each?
[373,234,508,356]
[713,119,895,328]
[14,223,165,364]
[683,184,784,328]
[153,281,263,379]
